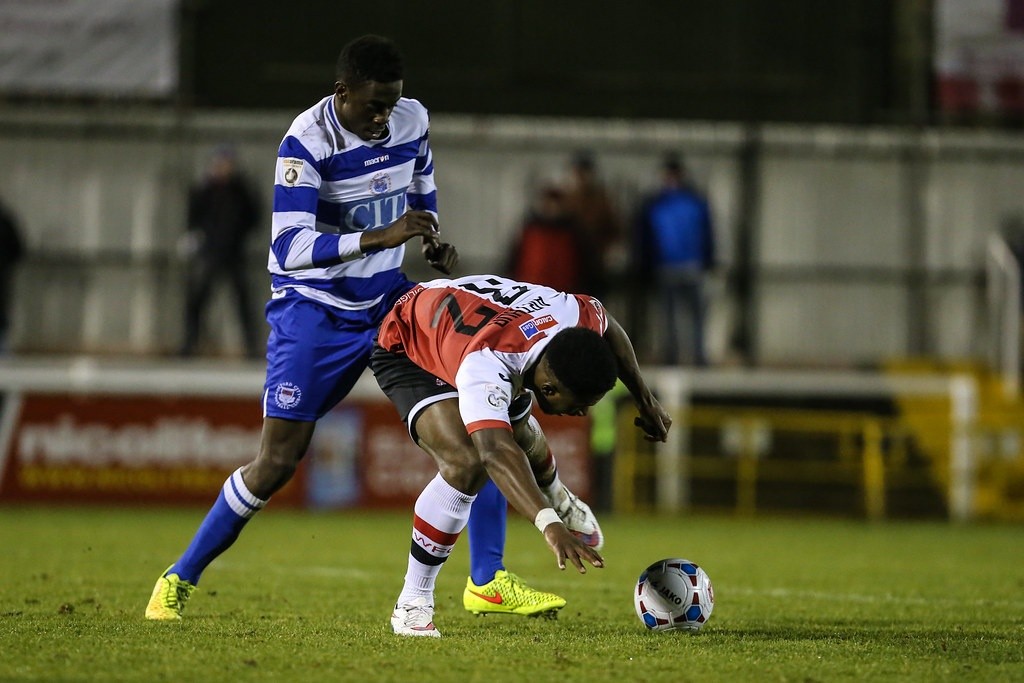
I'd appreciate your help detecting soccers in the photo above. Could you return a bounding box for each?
[632,558,717,632]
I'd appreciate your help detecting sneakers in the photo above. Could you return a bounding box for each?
[544,483,604,551]
[145,563,199,621]
[391,597,442,638]
[463,569,567,621]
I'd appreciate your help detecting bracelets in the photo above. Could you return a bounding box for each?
[535,508,564,534]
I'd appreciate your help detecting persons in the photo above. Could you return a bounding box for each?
[371,274,673,637]
[504,143,717,364]
[145,38,567,621]
[176,147,266,358]
[0,200,24,334]
